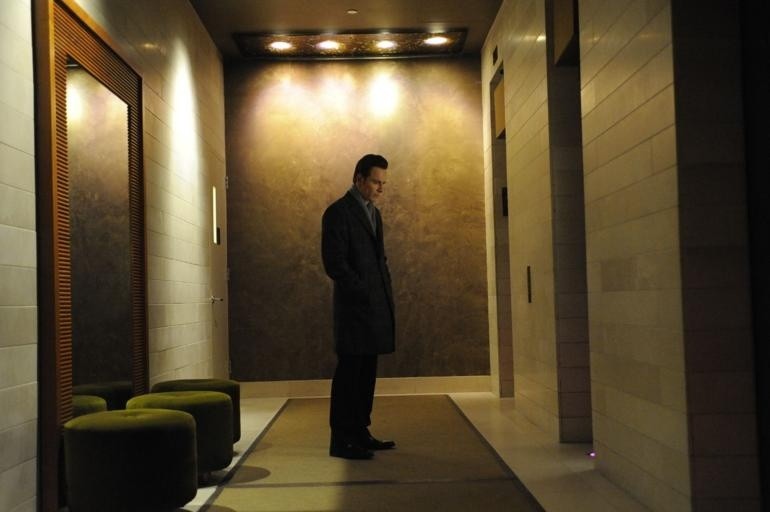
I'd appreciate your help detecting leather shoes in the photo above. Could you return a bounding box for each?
[330,444,374,459]
[353,436,395,449]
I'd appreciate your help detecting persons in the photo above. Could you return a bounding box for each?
[322,154,396,460]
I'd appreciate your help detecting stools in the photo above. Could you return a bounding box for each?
[151,379,240,443]
[126,391,233,482]
[72,395,107,415]
[63,409,197,512]
[72,381,133,410]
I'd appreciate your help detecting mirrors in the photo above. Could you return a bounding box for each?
[66,53,133,418]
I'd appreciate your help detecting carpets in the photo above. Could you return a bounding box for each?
[197,395,546,511]
